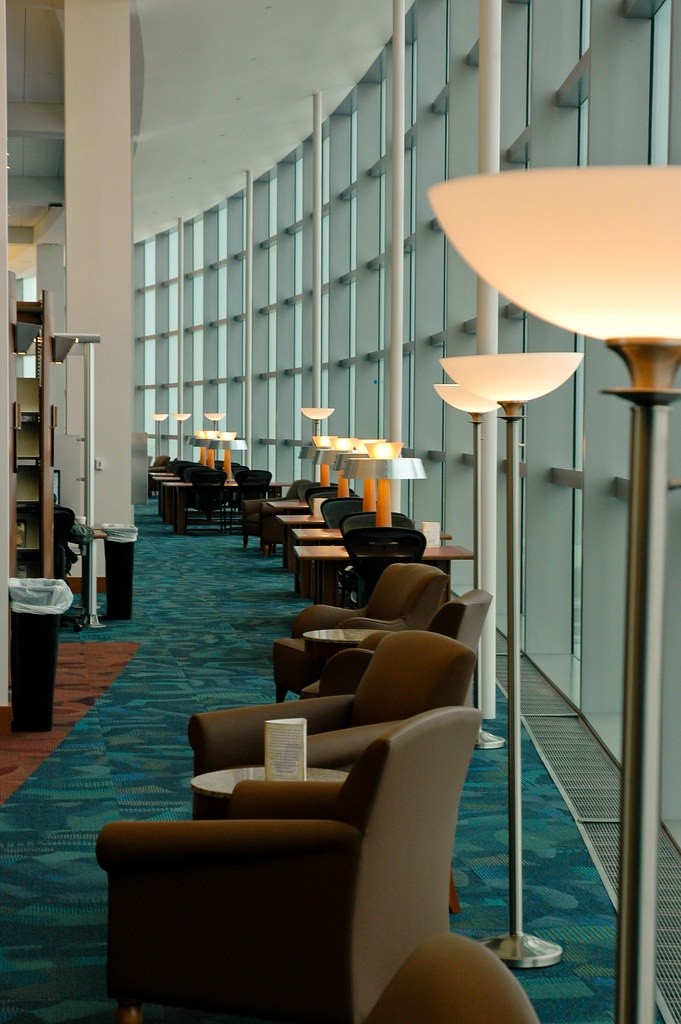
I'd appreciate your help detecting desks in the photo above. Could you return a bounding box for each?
[148,472,174,498]
[291,528,452,598]
[152,477,238,524]
[274,515,326,571]
[265,501,309,567]
[292,546,474,605]
[161,482,291,535]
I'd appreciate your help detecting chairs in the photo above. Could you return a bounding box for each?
[52,505,86,632]
[165,460,271,536]
[297,483,426,610]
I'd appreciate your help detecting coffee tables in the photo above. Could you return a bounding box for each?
[303,630,377,644]
[191,767,351,799]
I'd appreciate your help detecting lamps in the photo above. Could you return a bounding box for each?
[300,407,335,435]
[13,321,41,355]
[426,166,681,1024]
[333,439,388,512]
[438,353,585,971]
[298,436,338,487]
[171,413,191,461]
[52,333,78,364]
[341,442,428,529]
[187,430,248,480]
[312,438,359,497]
[432,384,506,750]
[150,414,169,456]
[204,413,226,431]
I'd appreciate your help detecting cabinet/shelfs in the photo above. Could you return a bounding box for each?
[12,289,54,578]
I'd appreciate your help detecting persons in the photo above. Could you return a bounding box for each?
[54,493,94,628]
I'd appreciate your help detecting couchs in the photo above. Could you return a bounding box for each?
[273,563,449,703]
[148,456,171,493]
[96,704,481,1024]
[241,480,311,557]
[186,630,482,914]
[299,589,493,702]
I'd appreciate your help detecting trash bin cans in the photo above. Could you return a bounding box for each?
[102,522,138,620]
[7,577,65,732]
